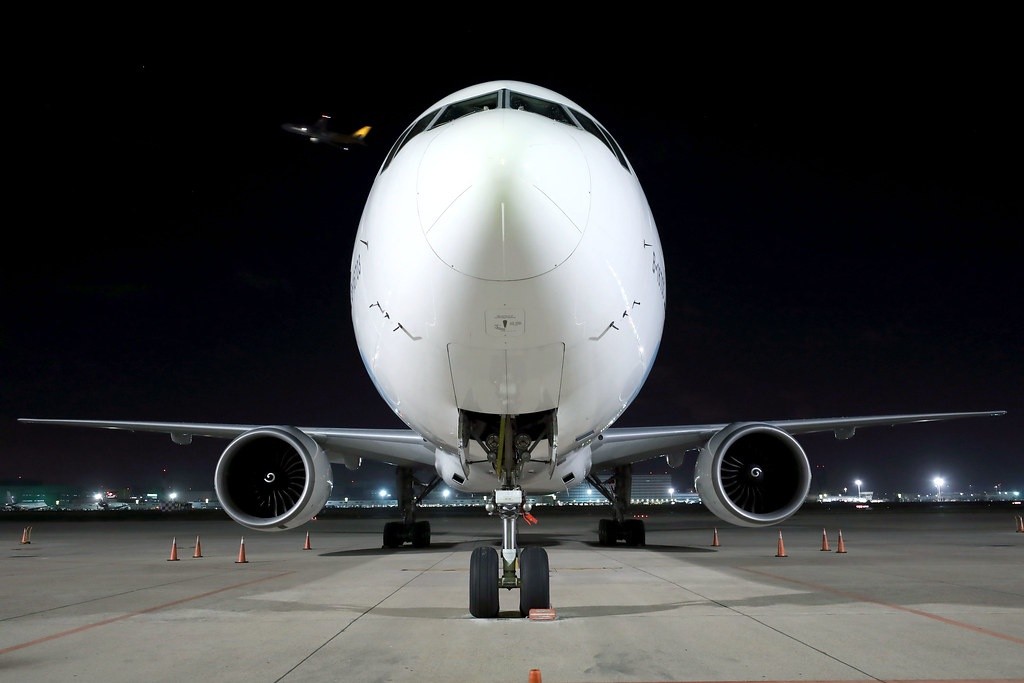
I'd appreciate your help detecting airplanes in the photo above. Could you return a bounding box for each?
[279,112,372,157]
[12,77,1007,620]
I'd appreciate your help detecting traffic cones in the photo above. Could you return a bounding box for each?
[774,528,788,558]
[233,535,249,563]
[20,526,28,545]
[834,528,848,553]
[164,536,180,561]
[26,526,32,544]
[710,527,721,547]
[1015,513,1024,532]
[300,530,311,550]
[190,535,203,558]
[819,527,831,551]
